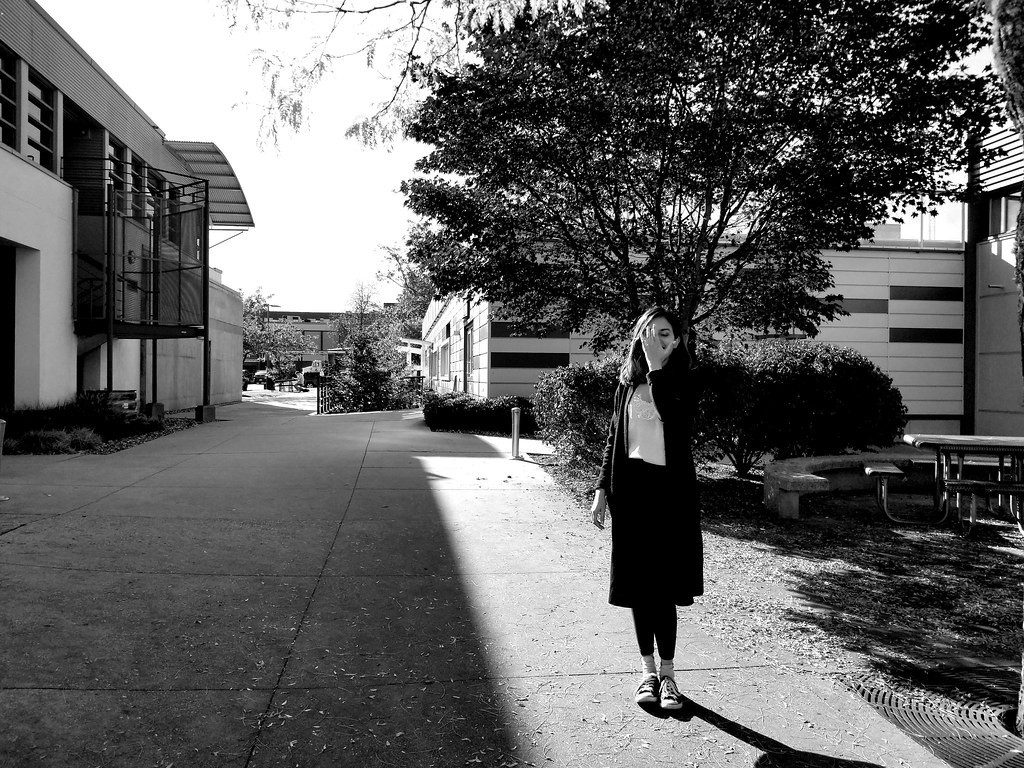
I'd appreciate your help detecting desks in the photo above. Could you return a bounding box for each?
[903,433,1024,525]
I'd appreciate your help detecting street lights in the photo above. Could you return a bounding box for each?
[267,302,281,389]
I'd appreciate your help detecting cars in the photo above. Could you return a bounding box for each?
[253,371,268,384]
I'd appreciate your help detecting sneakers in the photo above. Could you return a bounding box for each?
[633,673,659,704]
[659,676,683,710]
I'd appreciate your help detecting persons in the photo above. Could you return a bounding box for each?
[590,307,704,710]
[292,371,305,392]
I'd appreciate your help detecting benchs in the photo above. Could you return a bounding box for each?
[910,458,1017,510]
[943,479,1024,535]
[863,461,904,527]
